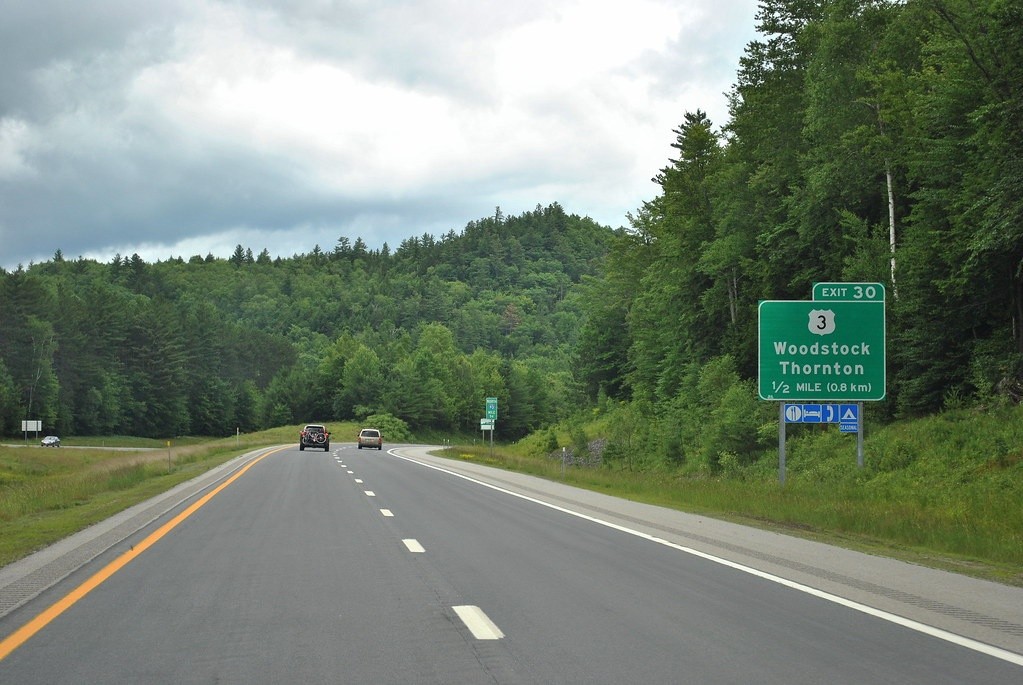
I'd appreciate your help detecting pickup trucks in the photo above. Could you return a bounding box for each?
[299,424,331,452]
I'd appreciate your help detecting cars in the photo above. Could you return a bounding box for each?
[357,428,384,450]
[41,435,60,447]
